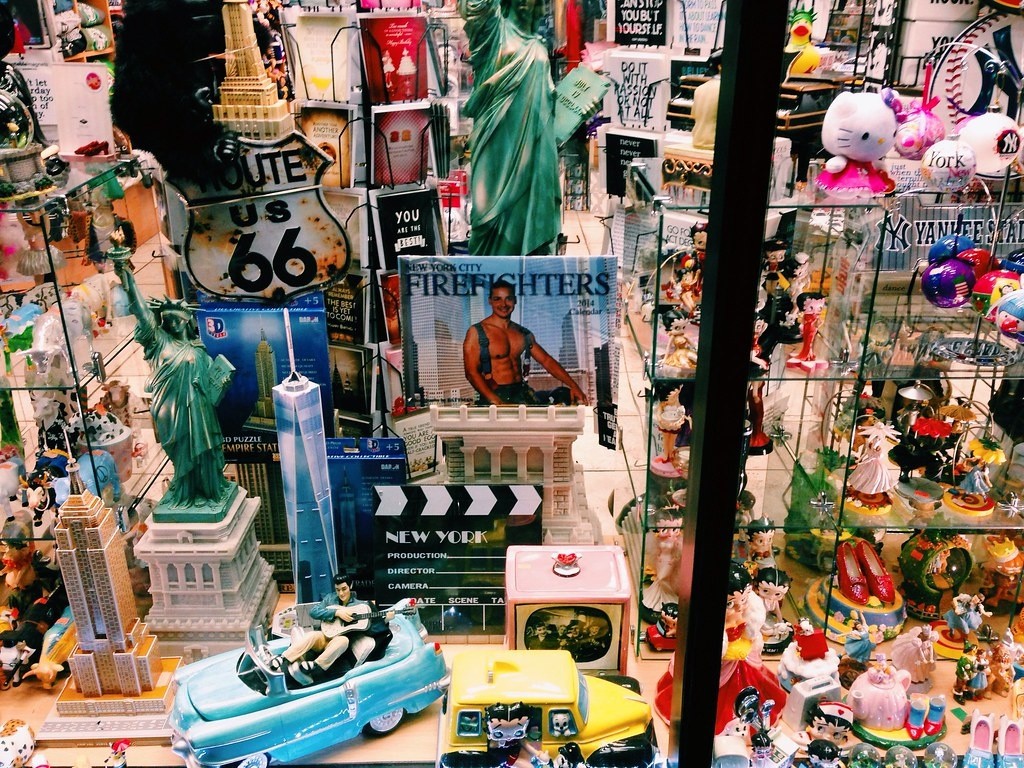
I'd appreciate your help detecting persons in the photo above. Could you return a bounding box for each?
[462,279,589,408]
[690,49,726,152]
[1,0,1024,768]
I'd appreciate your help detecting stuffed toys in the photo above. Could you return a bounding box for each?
[814,86,908,200]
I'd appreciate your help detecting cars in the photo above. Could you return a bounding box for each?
[441,646,657,768]
[167,597,452,767]
